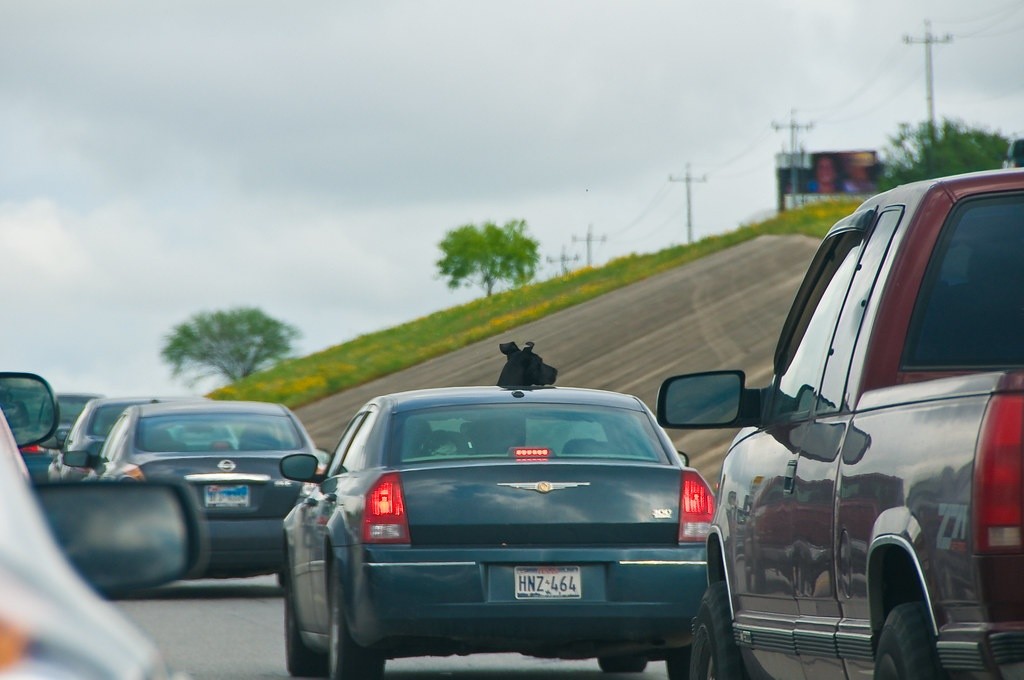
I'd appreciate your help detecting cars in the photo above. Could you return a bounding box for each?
[281,386,717,679]
[48,399,206,479]
[0,371,173,680]
[64,401,326,599]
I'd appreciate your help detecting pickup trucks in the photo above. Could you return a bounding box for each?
[656,167,1024,680]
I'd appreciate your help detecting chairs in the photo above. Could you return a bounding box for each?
[238,428,283,449]
[144,427,187,450]
[563,438,618,455]
[421,430,475,455]
